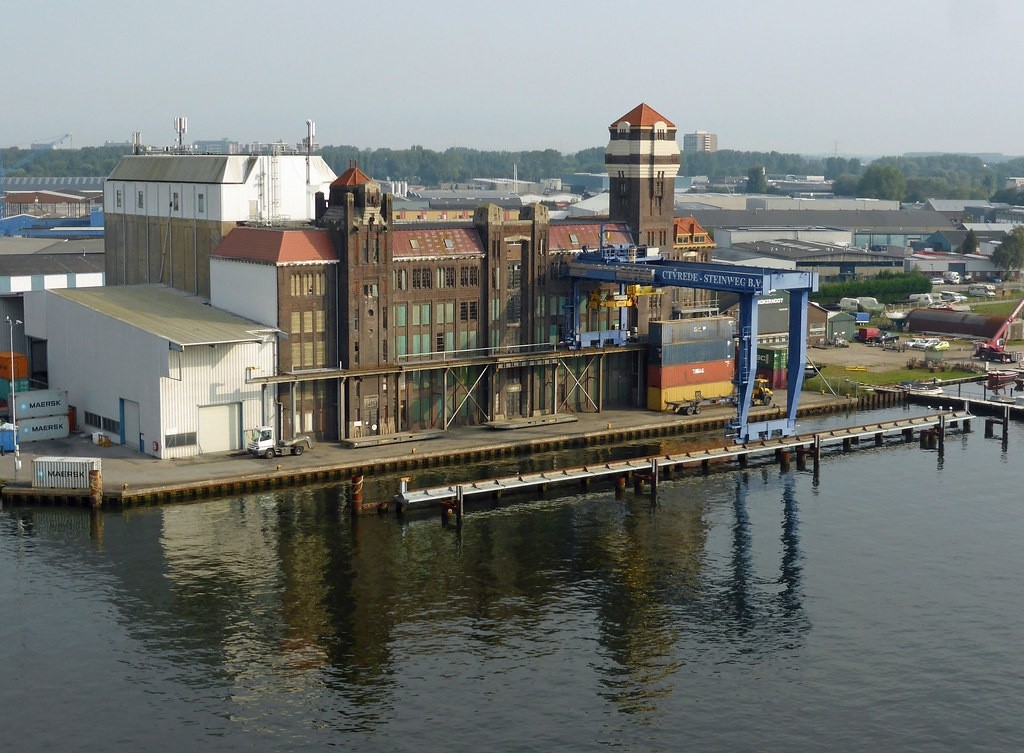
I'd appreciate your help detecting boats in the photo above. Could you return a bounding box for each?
[987,371,1019,382]
[897,378,943,395]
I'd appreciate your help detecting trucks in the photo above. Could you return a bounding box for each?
[942,271,960,284]
[969,284,996,297]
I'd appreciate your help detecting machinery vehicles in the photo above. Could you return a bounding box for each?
[247,426,315,459]
[973,299,1024,364]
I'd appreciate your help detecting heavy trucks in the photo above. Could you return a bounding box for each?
[664,378,774,415]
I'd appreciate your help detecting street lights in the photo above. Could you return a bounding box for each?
[4,316,22,480]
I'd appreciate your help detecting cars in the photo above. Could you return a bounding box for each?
[931,278,944,285]
[873,332,900,345]
[992,277,1002,283]
[903,337,923,348]
[917,338,940,350]
[942,291,967,302]
[930,341,949,351]
